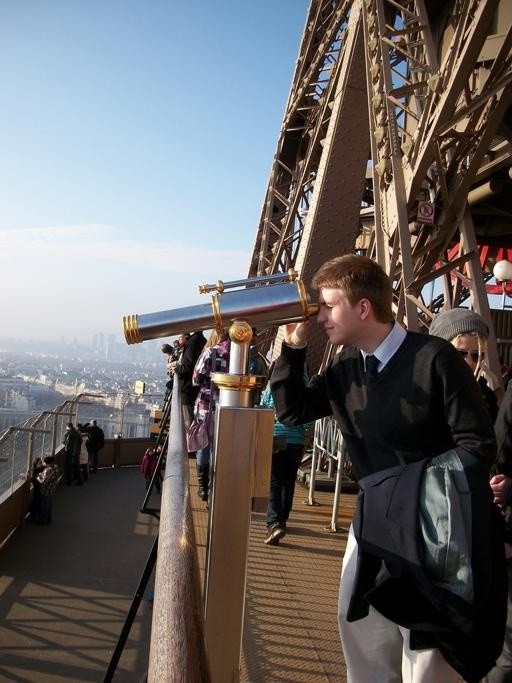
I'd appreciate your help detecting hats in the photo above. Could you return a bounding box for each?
[44,457,54,464]
[429,308,488,342]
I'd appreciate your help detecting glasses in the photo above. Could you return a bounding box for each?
[458,351,486,362]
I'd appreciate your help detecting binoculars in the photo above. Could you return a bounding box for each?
[123,268,320,345]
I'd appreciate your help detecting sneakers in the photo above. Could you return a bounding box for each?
[264,522,286,544]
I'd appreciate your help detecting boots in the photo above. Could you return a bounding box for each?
[196,462,208,500]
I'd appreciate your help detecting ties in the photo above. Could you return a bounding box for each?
[365,355,379,380]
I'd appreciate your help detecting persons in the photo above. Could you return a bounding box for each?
[190,323,230,500]
[26,456,44,525]
[36,456,64,524]
[61,419,105,486]
[270,254,496,683]
[481,356,512,683]
[425,306,512,508]
[153,444,164,482]
[159,329,207,458]
[140,447,157,496]
[260,353,315,545]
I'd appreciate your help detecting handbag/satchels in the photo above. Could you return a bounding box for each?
[187,413,213,453]
